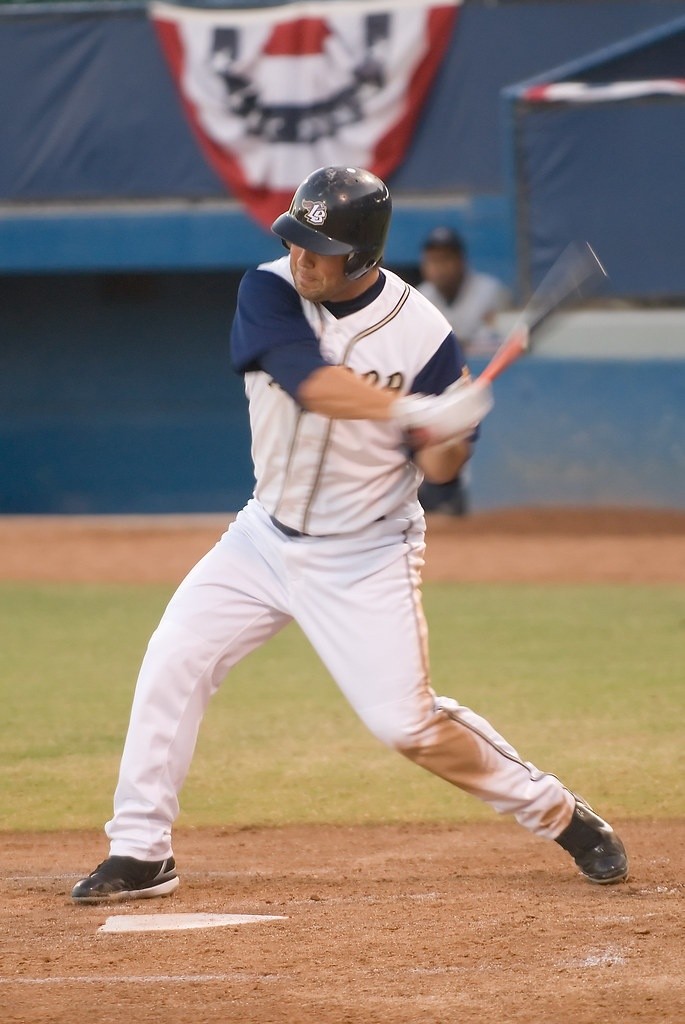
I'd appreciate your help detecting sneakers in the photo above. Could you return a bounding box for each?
[554,796,628,880]
[70,855,176,902]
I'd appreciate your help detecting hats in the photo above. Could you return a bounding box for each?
[422,228,461,255]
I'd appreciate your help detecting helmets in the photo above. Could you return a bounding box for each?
[270,163,390,282]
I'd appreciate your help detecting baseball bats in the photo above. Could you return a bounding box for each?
[400,243,608,456]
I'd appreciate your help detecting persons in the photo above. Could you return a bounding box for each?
[411,226,507,516]
[68,166,630,903]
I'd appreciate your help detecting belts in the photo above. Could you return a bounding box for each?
[268,515,385,537]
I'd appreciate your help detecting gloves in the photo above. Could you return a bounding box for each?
[395,379,495,434]
